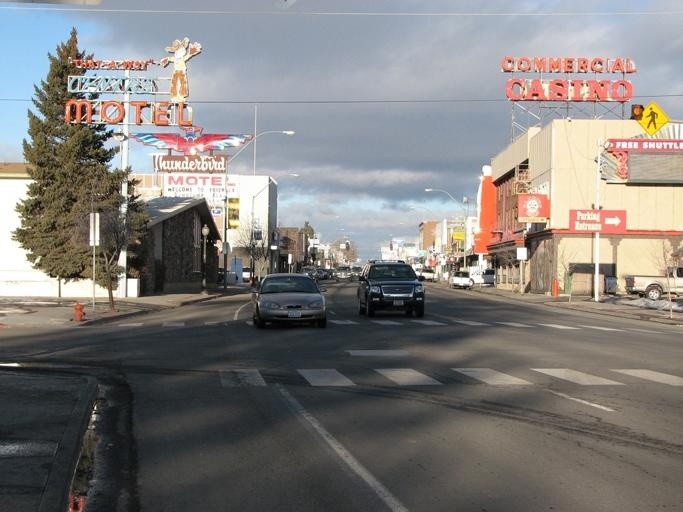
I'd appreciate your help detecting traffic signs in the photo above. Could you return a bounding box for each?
[568,209,626,235]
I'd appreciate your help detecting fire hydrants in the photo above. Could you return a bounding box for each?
[552,278,559,298]
[74,303,85,321]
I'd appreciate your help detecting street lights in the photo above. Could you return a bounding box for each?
[251,173,298,286]
[201,223,210,288]
[223,130,295,290]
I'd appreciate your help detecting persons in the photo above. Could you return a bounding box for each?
[159,37,203,97]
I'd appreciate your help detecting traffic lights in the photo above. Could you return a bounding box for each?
[630,105,644,120]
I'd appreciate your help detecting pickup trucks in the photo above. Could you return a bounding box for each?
[625,266,682,300]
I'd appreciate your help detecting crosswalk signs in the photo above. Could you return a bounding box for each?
[635,101,668,135]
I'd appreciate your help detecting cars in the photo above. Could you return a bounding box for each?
[448,271,473,289]
[414,268,437,282]
[252,272,326,328]
[357,259,424,318]
[299,265,361,282]
